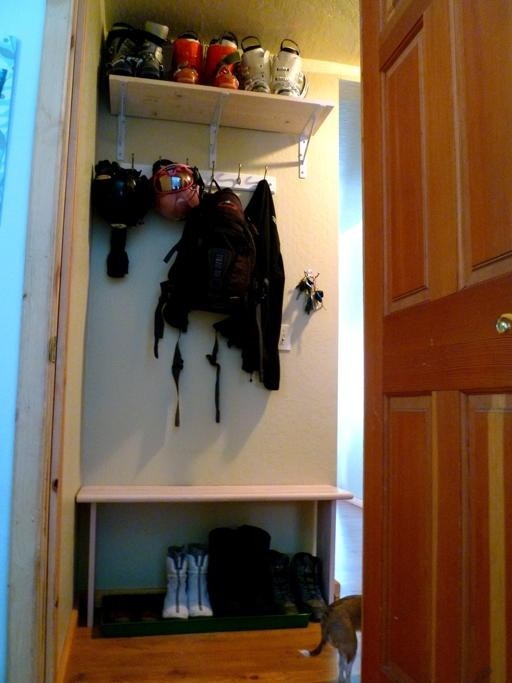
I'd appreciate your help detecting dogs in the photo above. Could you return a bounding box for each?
[297,594,362,683]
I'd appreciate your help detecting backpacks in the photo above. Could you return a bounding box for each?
[153,176,257,427]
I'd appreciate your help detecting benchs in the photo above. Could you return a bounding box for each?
[76,483,354,641]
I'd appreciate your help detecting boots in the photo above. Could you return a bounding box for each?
[162,523,327,628]
[102,20,308,99]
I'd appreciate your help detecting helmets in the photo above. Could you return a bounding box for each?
[150,159,204,223]
